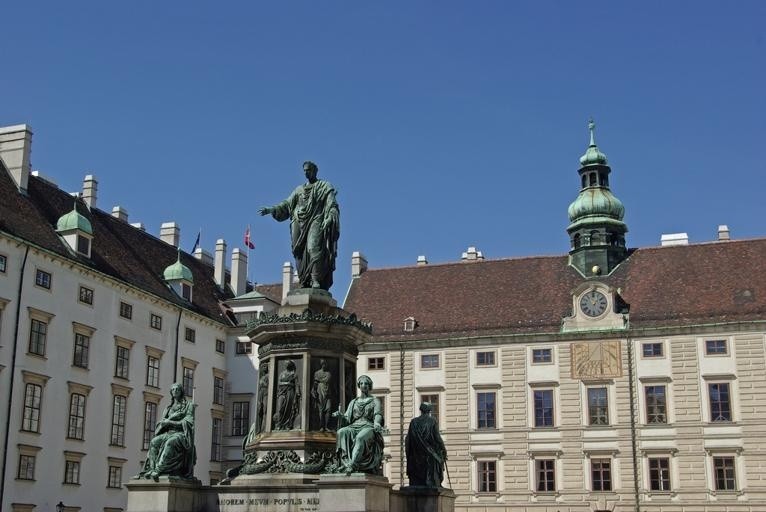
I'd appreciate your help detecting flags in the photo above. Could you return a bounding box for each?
[190,232,200,254]
[244,224,256,249]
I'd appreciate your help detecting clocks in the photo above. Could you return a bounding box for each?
[579,290,609,318]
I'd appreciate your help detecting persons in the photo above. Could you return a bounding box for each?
[404,401,447,486]
[257,160,341,291]
[257,362,269,434]
[273,359,301,430]
[310,358,333,433]
[139,382,198,480]
[330,375,385,476]
